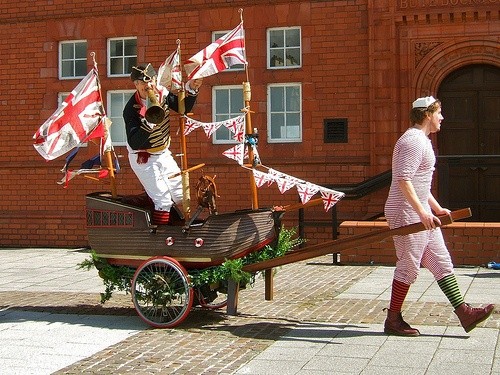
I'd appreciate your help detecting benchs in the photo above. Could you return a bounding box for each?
[85,191,284,273]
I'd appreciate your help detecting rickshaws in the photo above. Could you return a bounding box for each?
[83,189,474,329]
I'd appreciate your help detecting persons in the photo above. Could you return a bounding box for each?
[123,63,203,224]
[383,97,494,337]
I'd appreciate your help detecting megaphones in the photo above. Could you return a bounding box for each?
[143,85,166,125]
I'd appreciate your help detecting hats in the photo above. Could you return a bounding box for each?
[412,96,436,108]
[130,62,156,83]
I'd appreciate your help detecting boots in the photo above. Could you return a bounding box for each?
[453,303,495,333]
[382,307,420,336]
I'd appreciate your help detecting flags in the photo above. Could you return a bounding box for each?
[184,23,247,79]
[156,49,181,90]
[33,67,103,162]
